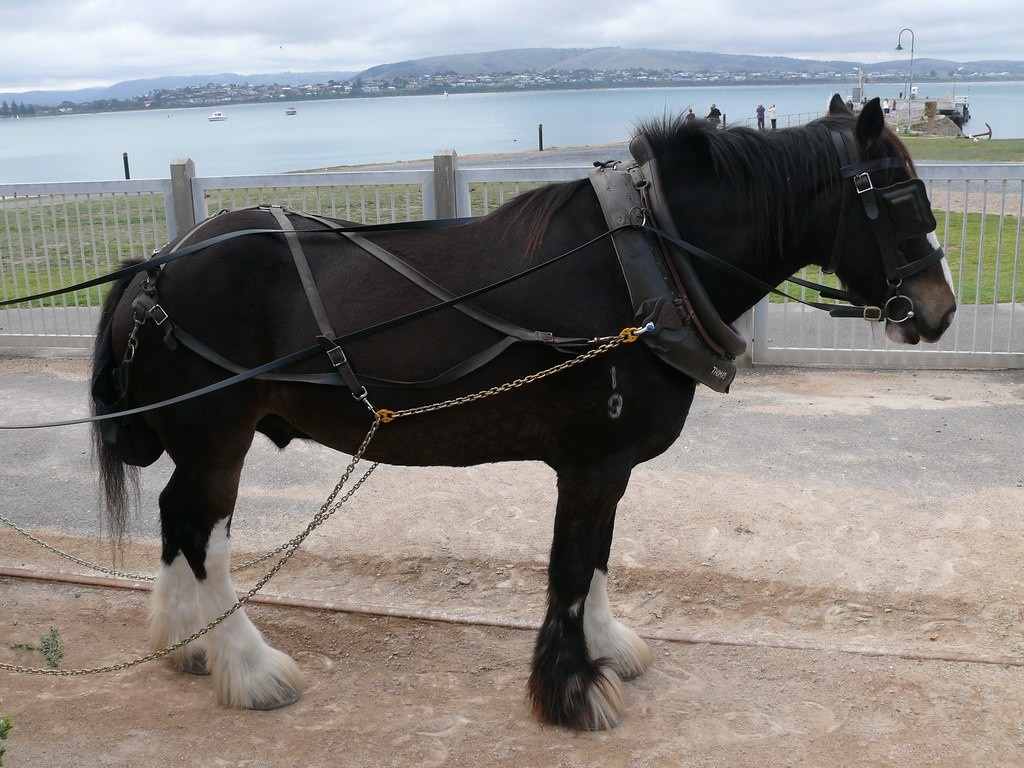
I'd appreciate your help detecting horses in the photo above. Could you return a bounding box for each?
[85,97,958,736]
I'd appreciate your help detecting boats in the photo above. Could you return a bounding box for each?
[285,106,296,115]
[208,112,228,121]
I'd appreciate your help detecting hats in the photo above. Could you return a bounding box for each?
[758,103,763,107]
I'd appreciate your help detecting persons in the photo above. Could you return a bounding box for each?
[686,108,695,122]
[861,97,868,109]
[892,99,897,116]
[756,104,765,131]
[898,87,903,98]
[883,98,890,114]
[768,104,777,130]
[848,100,853,111]
[705,104,721,128]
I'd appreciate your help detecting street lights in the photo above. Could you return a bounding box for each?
[895,25,915,131]
